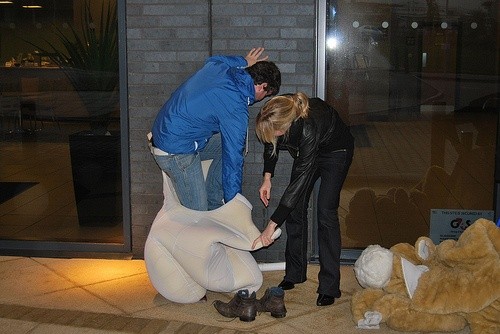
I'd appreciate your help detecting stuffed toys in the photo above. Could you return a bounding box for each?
[352,219,500,334]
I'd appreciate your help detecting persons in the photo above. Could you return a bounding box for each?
[250,91,355,305]
[148,48,281,211]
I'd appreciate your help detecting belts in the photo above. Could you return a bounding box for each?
[148,142,176,156]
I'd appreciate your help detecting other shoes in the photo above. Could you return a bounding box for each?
[316,293,341,306]
[279,279,307,290]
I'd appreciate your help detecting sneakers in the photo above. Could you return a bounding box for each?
[257,287,287,319]
[213,290,257,322]
